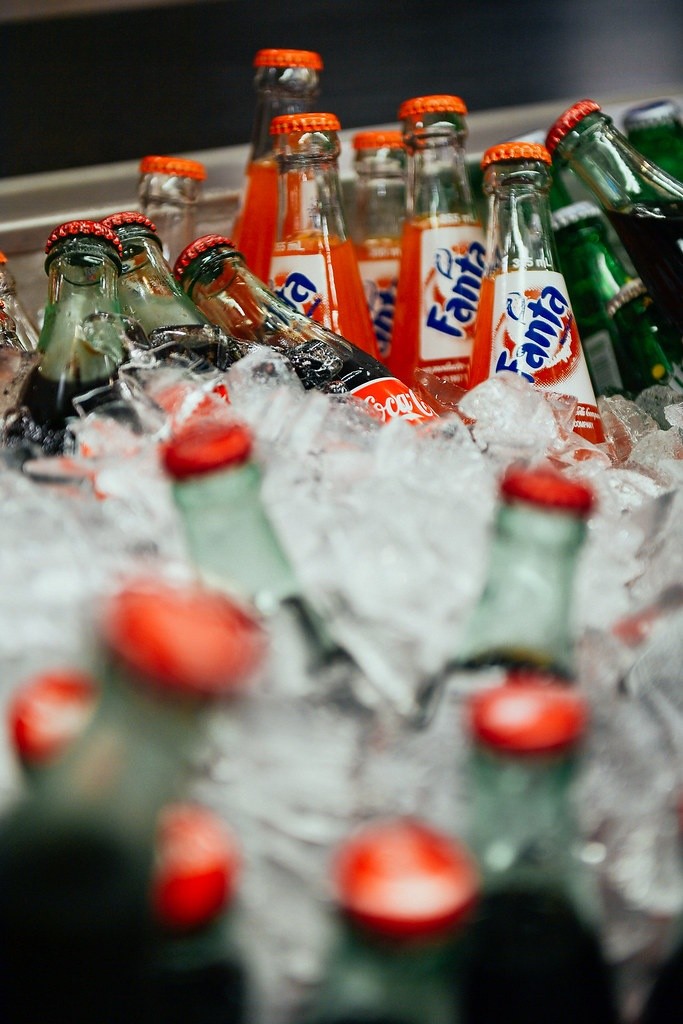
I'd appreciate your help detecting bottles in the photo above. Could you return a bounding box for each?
[0,47,683,1024]
